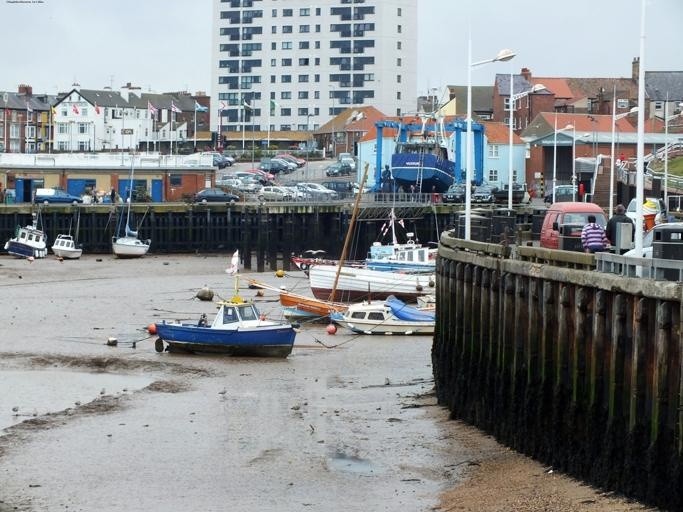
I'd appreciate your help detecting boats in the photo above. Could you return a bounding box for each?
[49,207,85,259]
[3,205,52,259]
[386,103,463,195]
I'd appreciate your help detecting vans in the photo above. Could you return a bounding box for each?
[33,187,84,206]
[540,194,683,282]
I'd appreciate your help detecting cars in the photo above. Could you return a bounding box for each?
[439,182,579,208]
[185,150,361,205]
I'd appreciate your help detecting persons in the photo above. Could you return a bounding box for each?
[91,186,98,204]
[581,212,611,254]
[109,186,114,206]
[605,203,635,271]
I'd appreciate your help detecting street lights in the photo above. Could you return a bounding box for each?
[505,70,547,216]
[550,111,575,205]
[326,84,337,159]
[660,91,683,217]
[570,120,596,204]
[607,83,638,218]
[462,30,517,247]
[305,113,316,163]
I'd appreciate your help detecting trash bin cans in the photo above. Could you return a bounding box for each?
[531,208,548,241]
[651,225,683,281]
[6,193,13,205]
[453,208,518,258]
[557,223,585,270]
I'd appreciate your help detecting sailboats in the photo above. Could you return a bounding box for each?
[239,162,386,322]
[104,142,153,257]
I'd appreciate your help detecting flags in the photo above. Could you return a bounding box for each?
[147,100,158,116]
[48,102,56,117]
[4,105,12,117]
[71,101,80,116]
[171,100,182,115]
[93,101,100,116]
[217,97,227,112]
[193,100,208,114]
[224,248,239,276]
[26,101,34,112]
